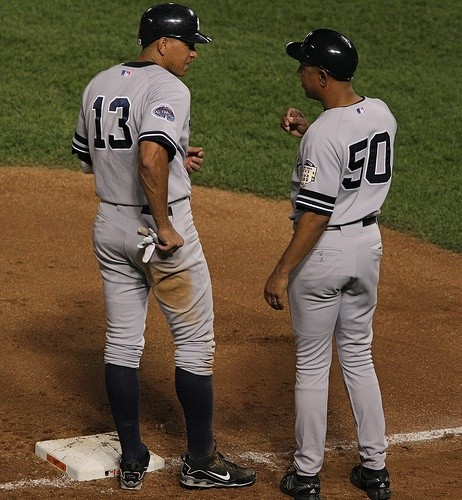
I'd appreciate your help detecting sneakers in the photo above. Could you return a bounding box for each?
[279,464,320,500]
[350,464,391,500]
[119,442,150,490]
[178,439,256,489]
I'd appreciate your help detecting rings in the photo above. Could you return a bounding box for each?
[272,300,278,306]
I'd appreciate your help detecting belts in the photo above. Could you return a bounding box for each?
[106,200,173,217]
[326,215,378,231]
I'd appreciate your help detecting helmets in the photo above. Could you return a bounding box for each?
[136,3,212,45]
[285,29,359,82]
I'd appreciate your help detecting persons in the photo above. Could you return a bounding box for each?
[264,29,396,500]
[72,3,257,491]
